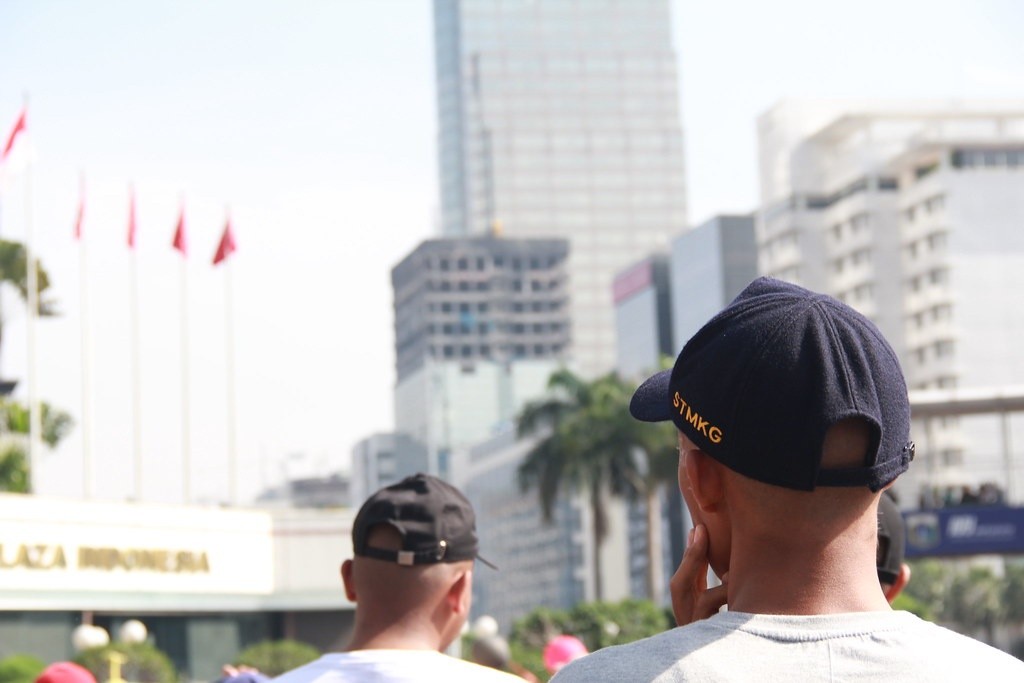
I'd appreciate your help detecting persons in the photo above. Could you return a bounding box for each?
[33,661,97,683]
[217,277,1024,682]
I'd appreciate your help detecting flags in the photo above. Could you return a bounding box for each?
[128,194,135,248]
[75,203,84,237]
[0,107,26,161]
[173,212,185,255]
[214,223,236,263]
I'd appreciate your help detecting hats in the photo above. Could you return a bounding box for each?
[353,473,500,570]
[630,274,916,492]
